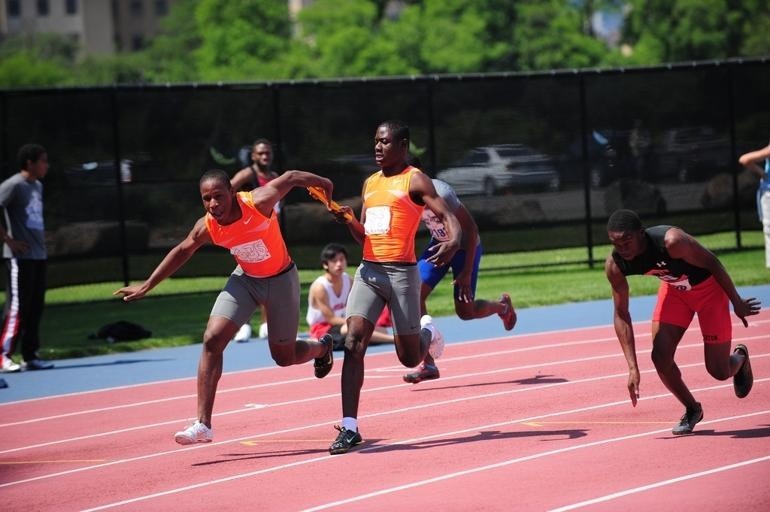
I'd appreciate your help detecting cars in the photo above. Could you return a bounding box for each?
[437,142,563,196]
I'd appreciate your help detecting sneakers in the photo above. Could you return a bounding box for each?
[421,314,444,359]
[1,354,21,371]
[498,291,517,331]
[234,324,252,343]
[175,419,213,445]
[731,345,754,398]
[329,424,362,455]
[402,362,440,383]
[20,358,52,370]
[258,322,267,339]
[314,335,334,378]
[672,403,702,434]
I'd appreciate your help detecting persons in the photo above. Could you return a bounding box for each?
[402,156,517,384]
[229,139,283,342]
[0,144,54,373]
[739,144,770,270]
[604,209,761,434]
[328,119,462,453]
[306,245,395,343]
[113,169,333,444]
